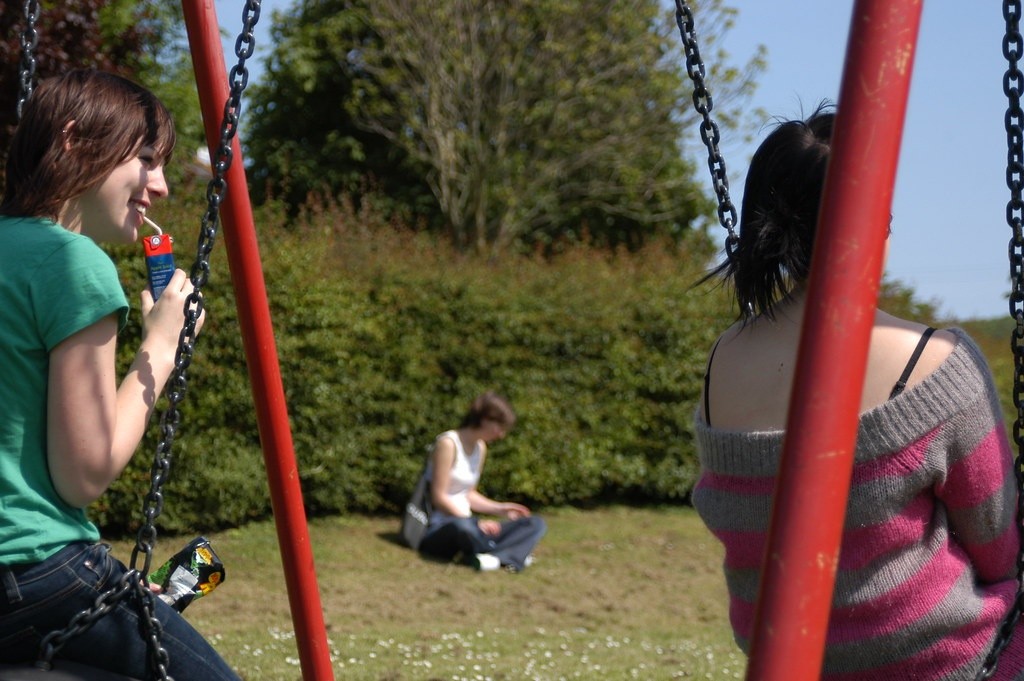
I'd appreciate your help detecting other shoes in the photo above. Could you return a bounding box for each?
[473,553,500,571]
[524,555,532,566]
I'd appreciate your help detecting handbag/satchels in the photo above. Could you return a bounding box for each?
[403,432,460,551]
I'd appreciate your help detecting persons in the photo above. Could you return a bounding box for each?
[693,105,1024,681]
[419,396,546,572]
[0,65,248,681]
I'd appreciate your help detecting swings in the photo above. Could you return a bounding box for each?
[0,0,267,681]
[677,0,1022,681]
